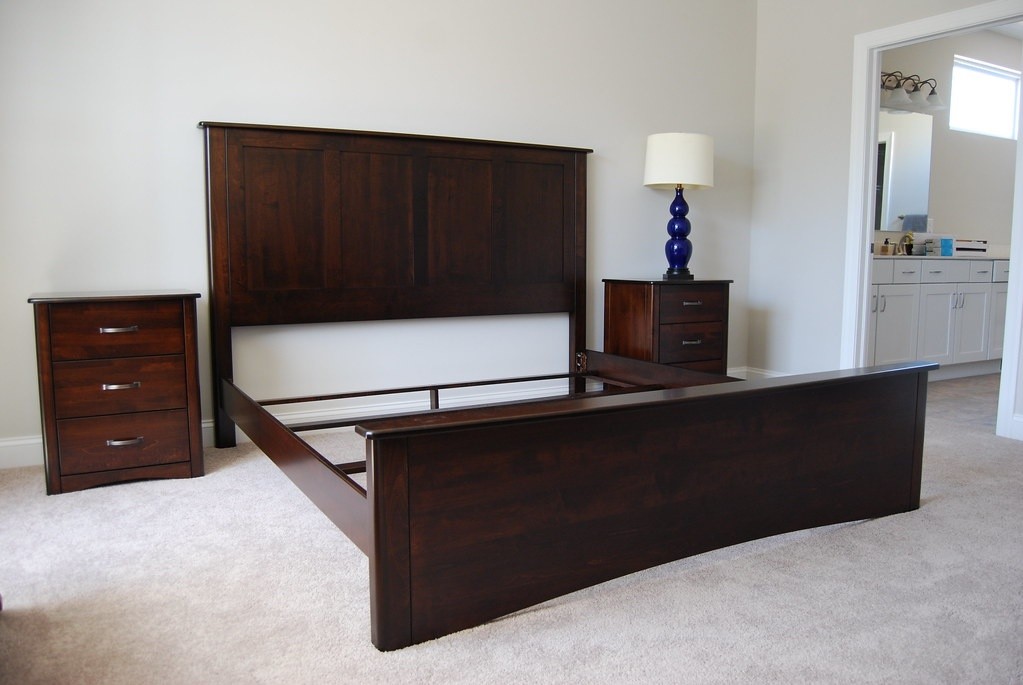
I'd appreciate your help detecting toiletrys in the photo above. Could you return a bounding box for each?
[881,238,890,255]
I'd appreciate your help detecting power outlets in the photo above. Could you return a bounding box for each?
[928,218,933,233]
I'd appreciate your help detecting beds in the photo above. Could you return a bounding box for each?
[197,120,942,654]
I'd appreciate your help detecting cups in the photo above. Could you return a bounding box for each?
[941,239,953,256]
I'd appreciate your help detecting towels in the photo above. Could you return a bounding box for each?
[902,215,927,233]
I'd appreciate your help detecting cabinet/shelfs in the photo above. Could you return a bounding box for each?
[27,291,205,497]
[601,278,734,391]
[916,259,993,382]
[868,259,922,366]
[986,259,1010,375]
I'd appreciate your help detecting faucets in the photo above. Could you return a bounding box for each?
[899,233,914,256]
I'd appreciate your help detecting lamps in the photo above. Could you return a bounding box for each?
[880,71,945,110]
[643,131,714,280]
